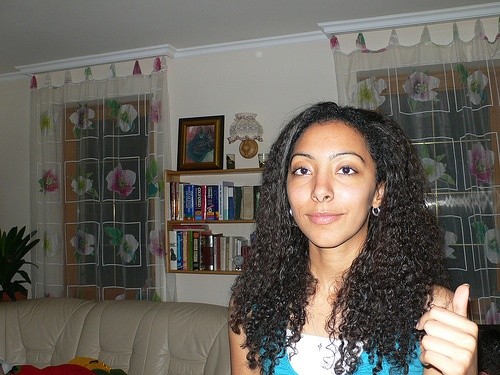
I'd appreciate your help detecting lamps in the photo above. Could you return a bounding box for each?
[227,112,264,159]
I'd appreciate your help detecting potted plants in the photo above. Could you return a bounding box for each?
[0,225,40,302]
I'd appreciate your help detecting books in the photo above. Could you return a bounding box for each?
[165,181,262,271]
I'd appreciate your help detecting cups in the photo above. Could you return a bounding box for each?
[258,152,268,168]
[226,154,236,169]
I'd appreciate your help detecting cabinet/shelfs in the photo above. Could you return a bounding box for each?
[163,168,266,275]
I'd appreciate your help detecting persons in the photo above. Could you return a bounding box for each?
[228,101,479,375]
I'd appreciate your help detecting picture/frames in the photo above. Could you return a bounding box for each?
[177,115,225,171]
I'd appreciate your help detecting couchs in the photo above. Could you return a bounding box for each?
[0,298,231,375]
